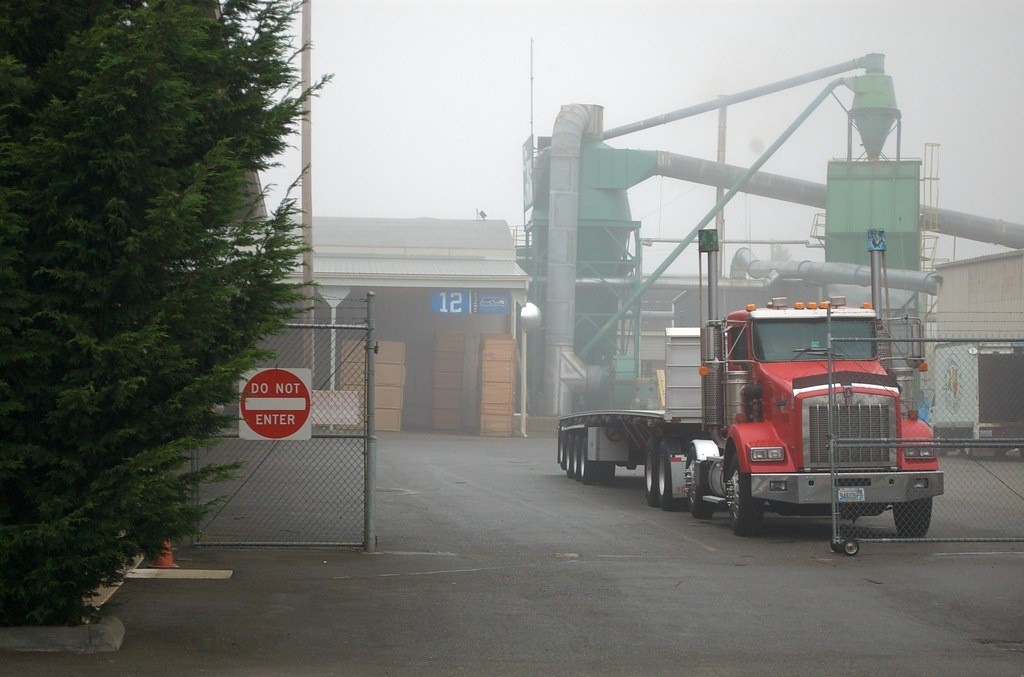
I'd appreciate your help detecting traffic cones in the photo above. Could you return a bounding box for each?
[147,538,179,569]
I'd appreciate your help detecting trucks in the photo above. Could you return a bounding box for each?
[932,342,1024,459]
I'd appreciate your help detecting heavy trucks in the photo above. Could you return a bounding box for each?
[557,229,944,539]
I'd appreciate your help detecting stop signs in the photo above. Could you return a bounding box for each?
[241,369,310,438]
